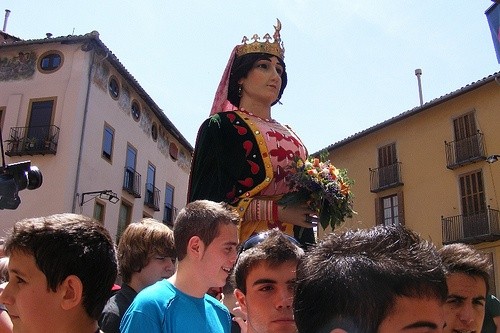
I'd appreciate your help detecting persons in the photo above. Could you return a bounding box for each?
[0,201,500,333]
[187,19,319,250]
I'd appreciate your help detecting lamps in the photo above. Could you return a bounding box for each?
[81,190,119,206]
[487,154,500,163]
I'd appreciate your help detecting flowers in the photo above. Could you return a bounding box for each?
[277,151,358,238]
